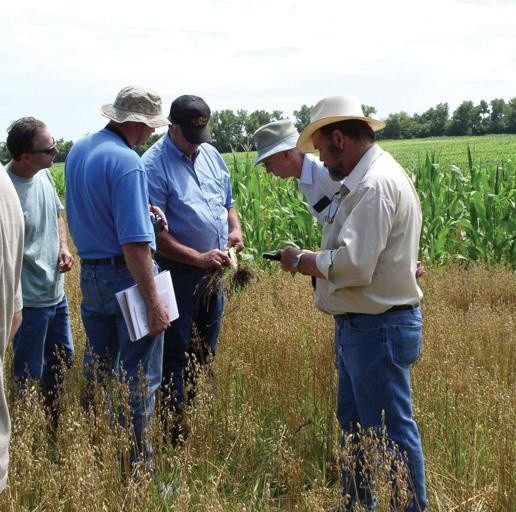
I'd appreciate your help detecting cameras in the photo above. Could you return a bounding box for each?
[149,212,164,234]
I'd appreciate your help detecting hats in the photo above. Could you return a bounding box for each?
[169,94,212,143]
[99,83,173,127]
[297,95,387,153]
[252,119,300,167]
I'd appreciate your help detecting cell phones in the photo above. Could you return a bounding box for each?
[263,253,281,260]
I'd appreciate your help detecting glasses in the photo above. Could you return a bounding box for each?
[29,137,57,155]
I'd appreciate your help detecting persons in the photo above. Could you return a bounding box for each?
[0,162,25,495]
[3,116,75,426]
[252,117,425,279]
[64,85,171,485]
[140,95,245,445]
[276,95,426,512]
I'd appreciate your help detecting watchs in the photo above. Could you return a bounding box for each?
[292,252,305,270]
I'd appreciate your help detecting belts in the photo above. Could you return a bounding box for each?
[80,254,124,268]
